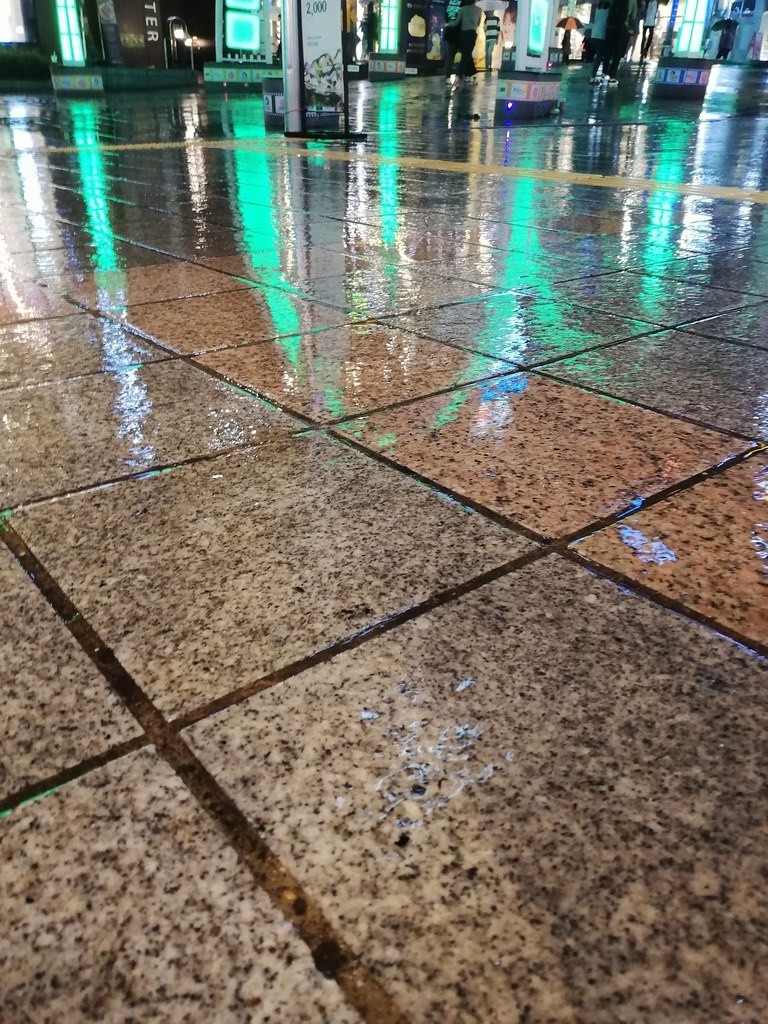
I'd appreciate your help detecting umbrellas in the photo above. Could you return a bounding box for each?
[578,23,594,35]
[556,16,583,29]
[476,0,505,12]
[712,18,739,30]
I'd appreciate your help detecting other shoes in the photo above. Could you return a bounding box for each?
[487,67,492,71]
[588,78,601,84]
[465,75,474,82]
[608,79,620,85]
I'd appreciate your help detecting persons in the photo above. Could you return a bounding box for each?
[562,29,571,64]
[359,2,379,56]
[444,0,480,85]
[503,1,517,49]
[713,27,739,60]
[483,10,500,72]
[582,0,658,88]
